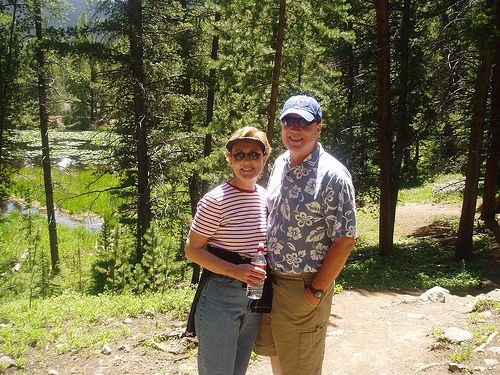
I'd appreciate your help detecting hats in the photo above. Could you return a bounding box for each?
[226,126,274,154]
[280,95,323,124]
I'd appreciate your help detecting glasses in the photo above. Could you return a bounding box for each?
[234,152,261,160]
[282,117,315,127]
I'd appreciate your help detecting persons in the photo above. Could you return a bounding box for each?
[184,126,271,375]
[252,95,356,375]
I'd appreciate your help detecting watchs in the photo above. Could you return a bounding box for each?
[309,284,325,299]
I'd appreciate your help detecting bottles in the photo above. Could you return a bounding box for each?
[246,245,268,299]
